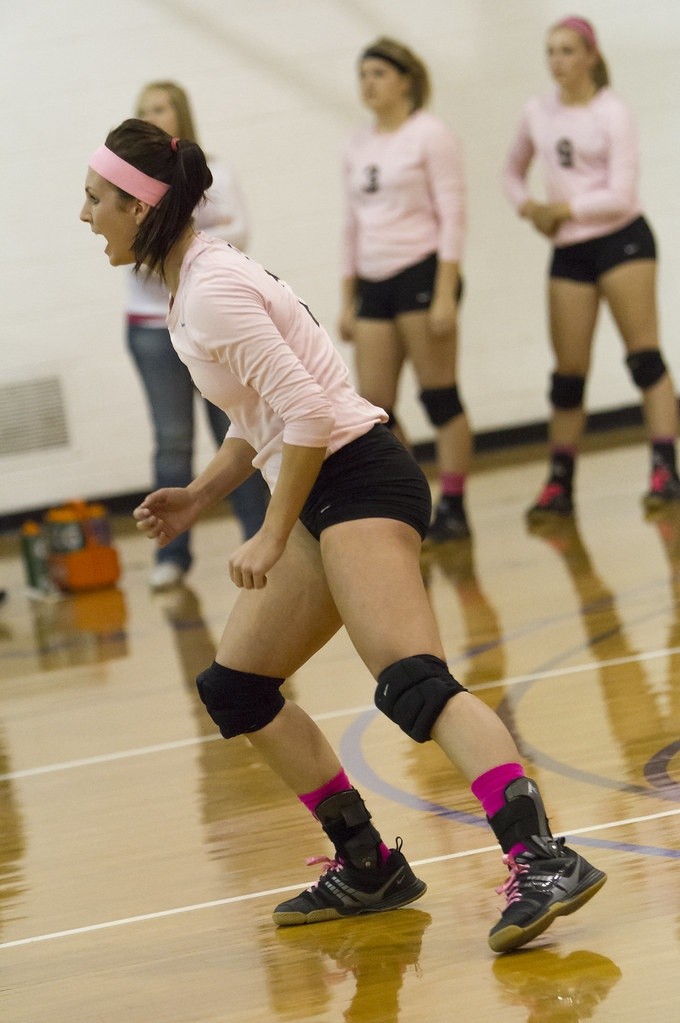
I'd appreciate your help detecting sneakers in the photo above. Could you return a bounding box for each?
[489,837,607,954]
[275,909,432,978]
[643,468,680,506]
[272,836,428,926]
[428,505,469,540]
[492,947,621,1003]
[522,484,571,524]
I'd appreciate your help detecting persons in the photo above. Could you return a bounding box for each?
[502,16,680,522]
[78,119,606,953]
[124,82,272,588]
[338,40,477,541]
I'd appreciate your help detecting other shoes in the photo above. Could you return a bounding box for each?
[151,562,181,590]
